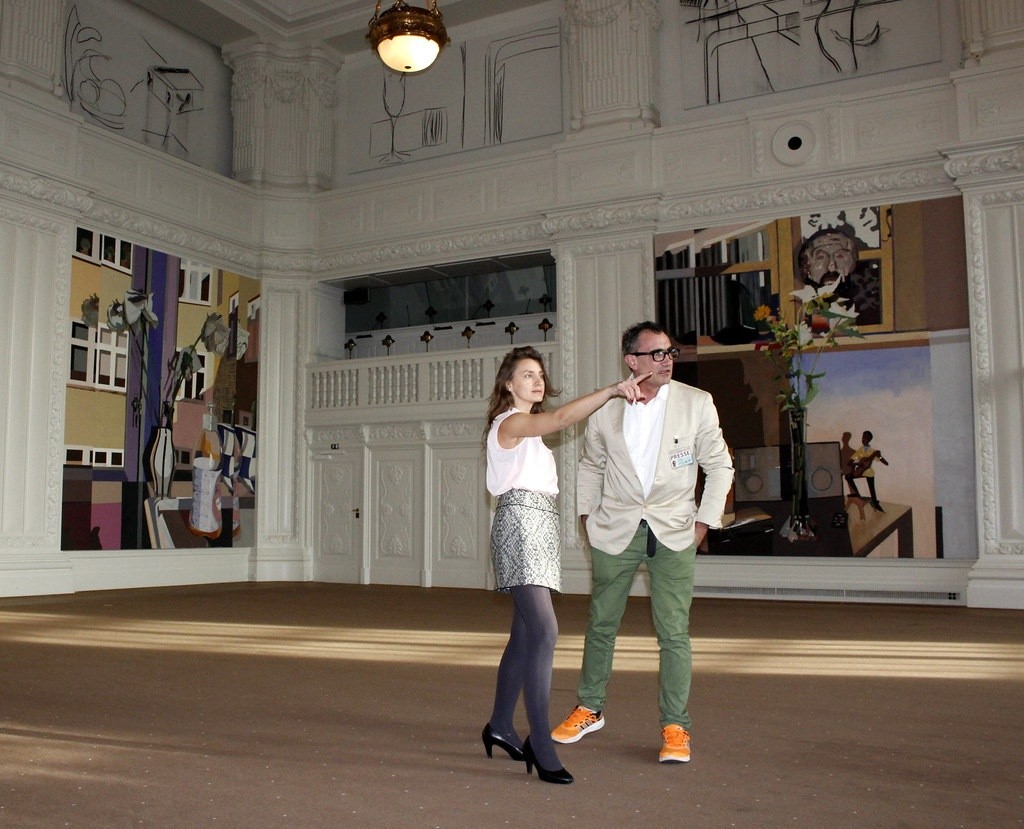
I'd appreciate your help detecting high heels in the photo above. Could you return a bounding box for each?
[482,722,574,785]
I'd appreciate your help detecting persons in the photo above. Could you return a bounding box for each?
[550,319,735,763]
[478,346,655,784]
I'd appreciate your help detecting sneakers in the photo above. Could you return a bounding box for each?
[658,724,692,764]
[550,704,606,744]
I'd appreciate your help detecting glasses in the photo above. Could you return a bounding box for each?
[628,347,681,362]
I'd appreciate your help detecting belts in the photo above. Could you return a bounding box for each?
[640,518,656,558]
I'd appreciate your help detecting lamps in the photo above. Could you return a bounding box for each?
[366,1,451,74]
